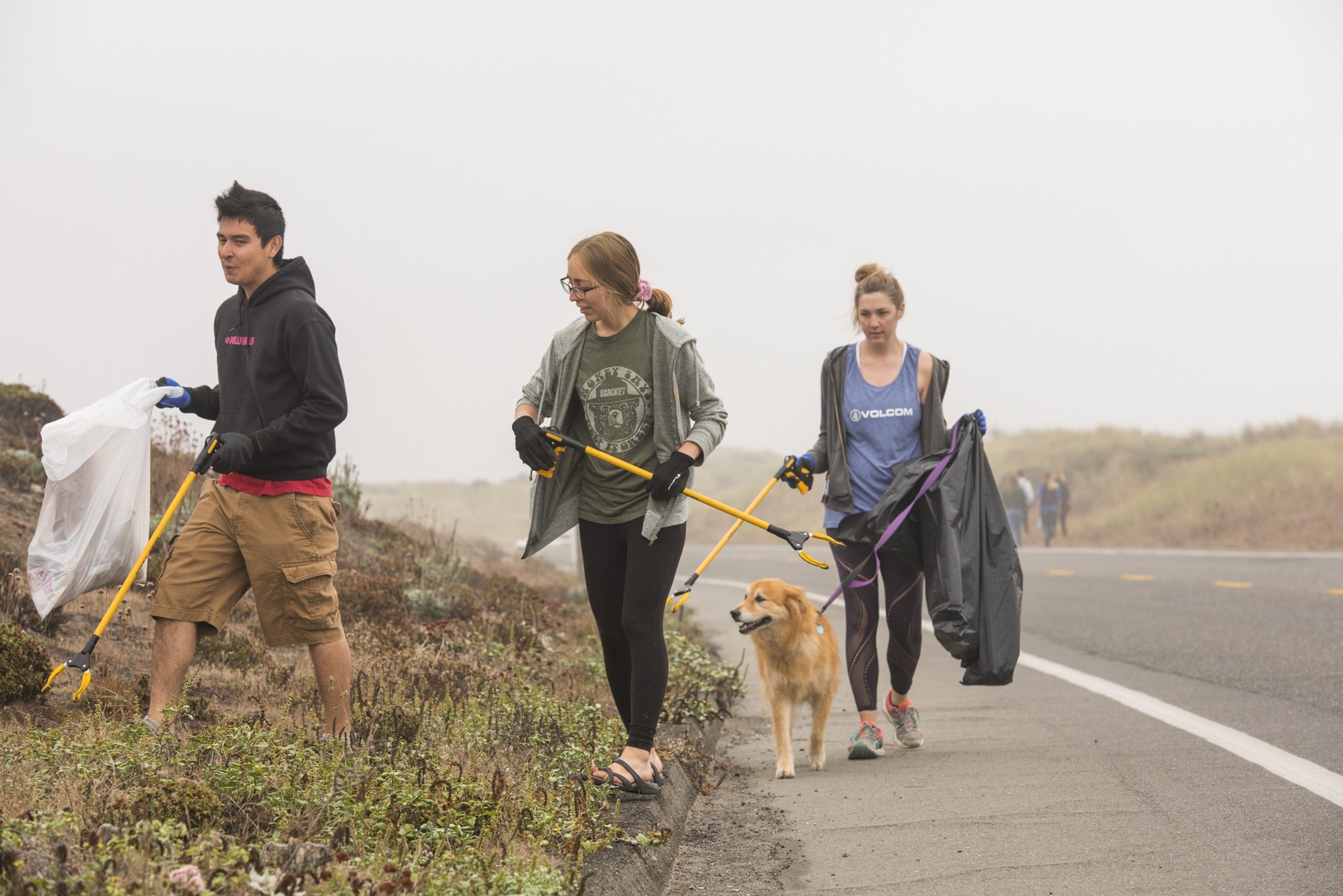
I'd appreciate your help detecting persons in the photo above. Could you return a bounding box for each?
[779,262,988,757]
[1016,469,1034,534]
[1054,469,1069,540]
[511,231,729,794]
[1003,477,1025,549]
[135,179,357,744]
[1039,470,1064,547]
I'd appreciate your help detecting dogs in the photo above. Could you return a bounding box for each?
[728,576,842,781]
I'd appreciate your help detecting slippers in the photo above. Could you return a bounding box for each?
[589,752,664,794]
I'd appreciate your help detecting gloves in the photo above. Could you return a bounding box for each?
[960,408,987,437]
[200,431,254,476]
[781,455,814,489]
[646,450,696,496]
[512,415,558,472]
[153,375,191,409]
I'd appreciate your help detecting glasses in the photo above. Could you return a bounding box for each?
[560,276,599,299]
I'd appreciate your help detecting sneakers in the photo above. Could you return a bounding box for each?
[882,690,924,750]
[848,720,884,759]
